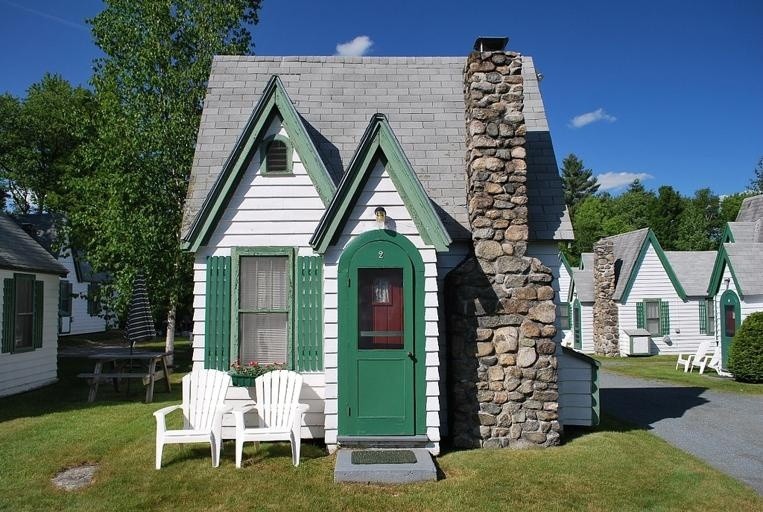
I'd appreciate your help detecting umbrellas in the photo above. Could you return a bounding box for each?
[117,271,157,391]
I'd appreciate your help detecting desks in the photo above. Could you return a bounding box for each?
[87,351,174,404]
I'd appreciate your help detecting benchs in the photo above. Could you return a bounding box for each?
[75,363,176,403]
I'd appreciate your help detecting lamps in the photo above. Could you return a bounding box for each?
[723,278,731,286]
[574,292,576,296]
[374,206,386,230]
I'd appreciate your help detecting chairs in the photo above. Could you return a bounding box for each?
[152,368,310,470]
[676,340,722,375]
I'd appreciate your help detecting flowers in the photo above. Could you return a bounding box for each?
[230,361,281,375]
